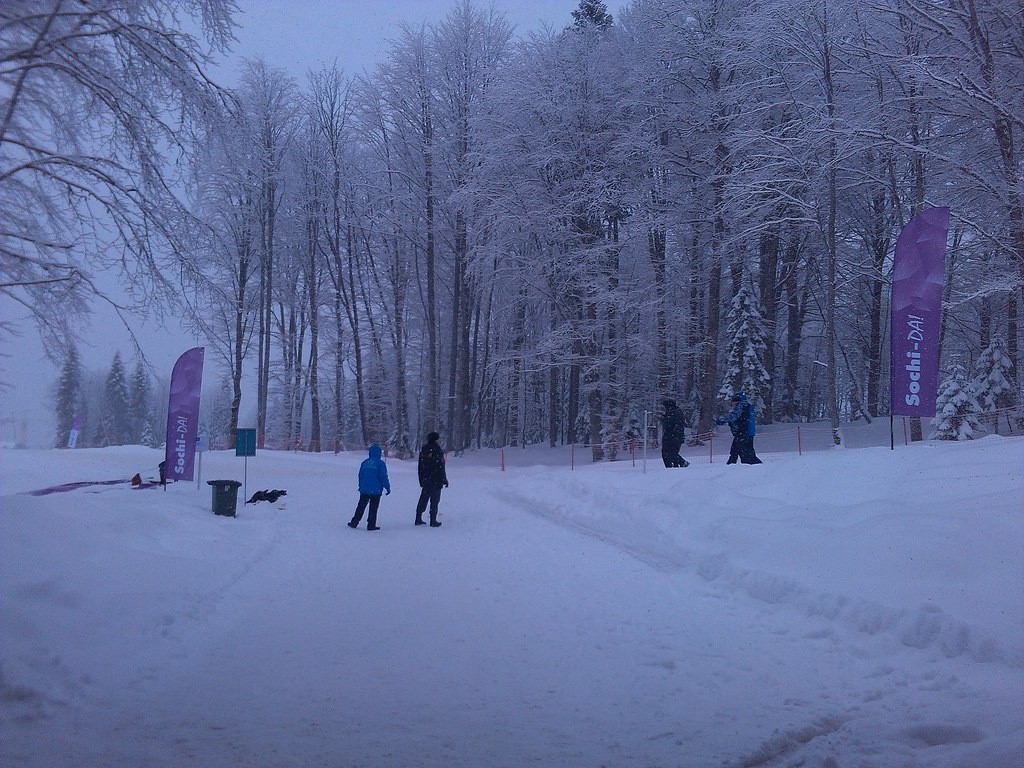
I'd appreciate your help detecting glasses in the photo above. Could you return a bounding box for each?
[438,437,441,439]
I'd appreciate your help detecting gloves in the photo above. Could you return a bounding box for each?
[442,480,448,488]
[712,417,723,426]
[358,488,360,493]
[385,488,390,496]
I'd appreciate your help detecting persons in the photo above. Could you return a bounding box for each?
[415,432,448,527]
[712,392,763,464]
[347,445,391,531]
[655,398,690,468]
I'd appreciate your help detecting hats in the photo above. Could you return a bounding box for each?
[734,392,747,402]
[427,432,439,443]
[663,399,676,406]
[731,395,741,402]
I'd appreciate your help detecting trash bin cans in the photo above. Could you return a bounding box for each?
[207,480,242,517]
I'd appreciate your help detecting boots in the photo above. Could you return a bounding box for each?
[415,511,426,526]
[430,514,442,527]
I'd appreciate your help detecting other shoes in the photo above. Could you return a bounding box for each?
[347,522,357,528]
[367,524,380,531]
[680,461,690,467]
